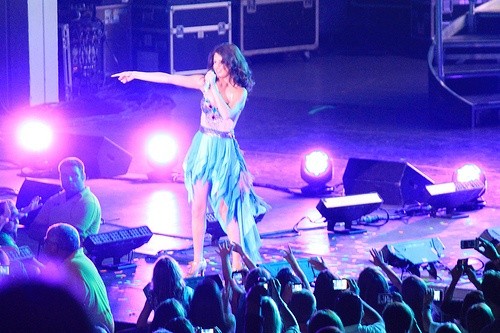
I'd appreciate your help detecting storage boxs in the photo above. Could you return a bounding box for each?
[232,0,320,56]
[136,1,232,74]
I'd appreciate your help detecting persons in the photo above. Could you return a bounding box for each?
[0,158,116,333]
[135,237,500,333]
[110,42,271,278]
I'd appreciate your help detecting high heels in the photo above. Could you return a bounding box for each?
[184,258,207,277]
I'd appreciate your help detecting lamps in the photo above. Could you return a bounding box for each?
[452,164,487,211]
[300,150,334,194]
[143,131,182,183]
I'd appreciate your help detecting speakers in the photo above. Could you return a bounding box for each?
[16,178,63,226]
[98,6,133,77]
[54,132,132,179]
[343,157,436,205]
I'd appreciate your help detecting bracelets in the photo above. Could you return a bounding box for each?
[1,214,9,222]
[242,254,247,260]
[21,208,28,217]
